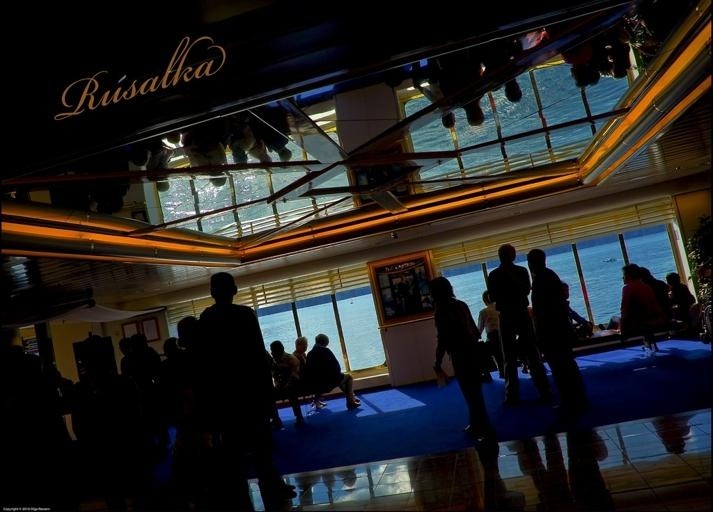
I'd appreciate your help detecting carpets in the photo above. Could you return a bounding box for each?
[71,337,712,484]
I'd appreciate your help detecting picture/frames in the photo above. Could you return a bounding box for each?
[366,249,436,326]
[120,317,161,343]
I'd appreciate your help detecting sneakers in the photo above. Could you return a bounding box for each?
[346,398,361,409]
[312,401,327,409]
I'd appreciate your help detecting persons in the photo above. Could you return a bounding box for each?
[476,290,504,379]
[488,242,553,410]
[6,99,289,215]
[470,413,693,510]
[426,276,498,452]
[526,248,589,420]
[559,280,594,342]
[619,263,661,339]
[666,272,695,317]
[414,14,634,126]
[637,267,672,329]
[1,272,361,511]
